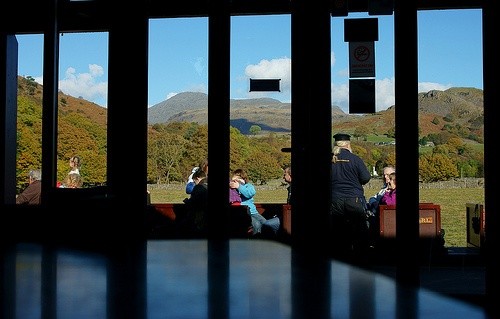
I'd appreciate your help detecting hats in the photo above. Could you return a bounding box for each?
[333,133,351,141]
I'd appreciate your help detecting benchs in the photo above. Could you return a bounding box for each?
[465,202,485,247]
[151,203,292,234]
[380,202,441,239]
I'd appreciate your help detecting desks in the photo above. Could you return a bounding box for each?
[0,238,486,318]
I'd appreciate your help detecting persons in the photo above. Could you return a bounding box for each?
[16,170,41,205]
[182,159,267,236]
[282,164,291,205]
[59,156,83,188]
[331,133,395,249]
